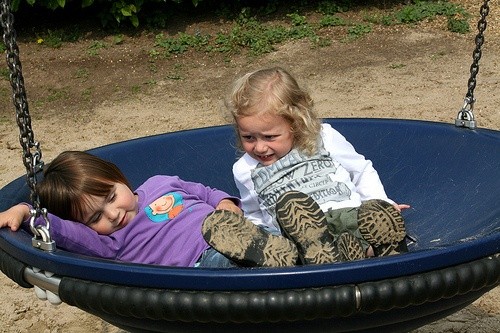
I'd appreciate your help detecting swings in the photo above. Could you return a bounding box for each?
[0,0,500,333]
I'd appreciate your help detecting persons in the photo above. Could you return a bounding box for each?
[0,150,412,269]
[203,65,415,267]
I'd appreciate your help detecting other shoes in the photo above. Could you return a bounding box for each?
[200,209,298,266]
[336,231,366,262]
[275,190,343,264]
[357,199,409,257]
